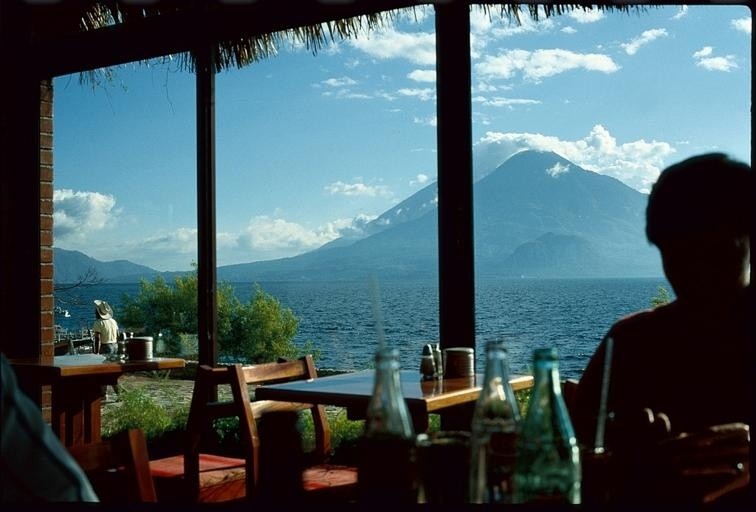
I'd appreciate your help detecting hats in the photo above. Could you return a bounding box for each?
[94,299,113,319]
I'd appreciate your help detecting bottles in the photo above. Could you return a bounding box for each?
[470,339,583,512]
[361,348,415,506]
[420,343,443,378]
[118,332,134,358]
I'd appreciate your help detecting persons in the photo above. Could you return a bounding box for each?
[558,154,752,511]
[91,299,124,403]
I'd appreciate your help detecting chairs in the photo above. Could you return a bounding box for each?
[227,355,359,512]
[149,358,289,512]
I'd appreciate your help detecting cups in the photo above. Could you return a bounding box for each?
[417,430,480,503]
